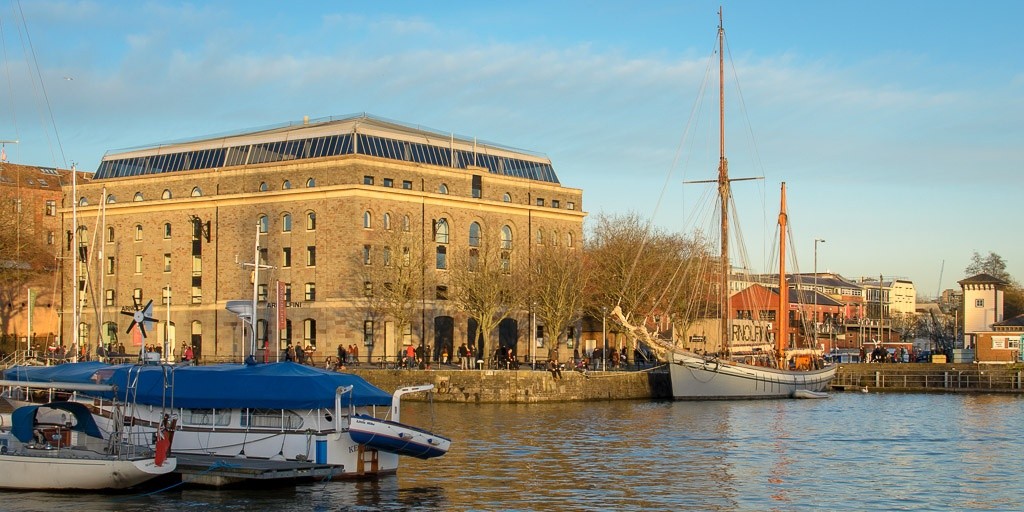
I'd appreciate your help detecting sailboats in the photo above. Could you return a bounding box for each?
[613,6,838,400]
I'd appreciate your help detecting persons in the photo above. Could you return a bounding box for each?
[0,339,201,367]
[399,335,653,383]
[858,343,923,363]
[282,338,361,368]
[685,346,732,359]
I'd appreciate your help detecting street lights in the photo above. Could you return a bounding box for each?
[814,239,826,348]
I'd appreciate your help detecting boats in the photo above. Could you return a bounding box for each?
[6,359,449,487]
[0,379,177,491]
